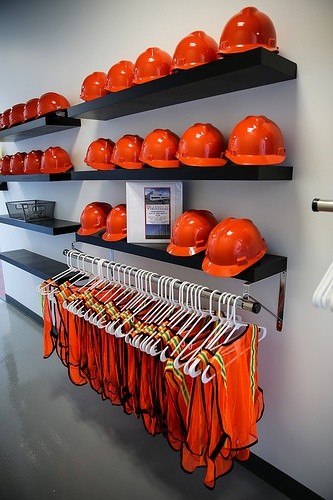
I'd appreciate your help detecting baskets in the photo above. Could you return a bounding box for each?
[6,200,55,222]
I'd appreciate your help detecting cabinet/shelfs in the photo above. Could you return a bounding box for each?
[0,47,297,283]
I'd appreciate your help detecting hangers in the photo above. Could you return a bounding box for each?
[36,248,268,384]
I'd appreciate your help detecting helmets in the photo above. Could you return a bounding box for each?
[80,72,109,101]
[102,204,126,241]
[175,123,228,166]
[166,209,218,256]
[77,202,113,235]
[225,115,287,165]
[36,92,71,117]
[110,134,145,169]
[40,146,73,174]
[202,217,268,278]
[217,7,280,58]
[0,98,39,130]
[132,47,173,84]
[138,129,181,168]
[105,60,134,92]
[171,31,223,71]
[0,150,44,175]
[84,138,118,170]
